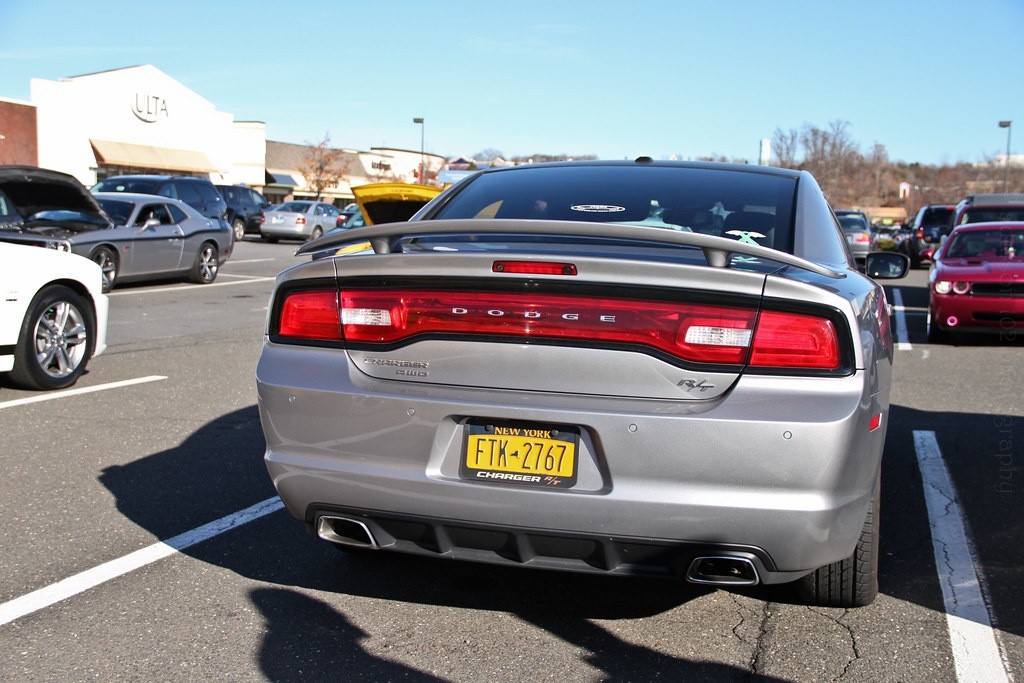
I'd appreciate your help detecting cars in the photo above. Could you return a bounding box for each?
[334,183,454,256]
[255,160,911,609]
[0,240,108,391]
[870,218,916,255]
[917,220,1024,345]
[835,209,875,260]
[89,172,228,220]
[215,185,273,242]
[312,212,369,259]
[259,201,340,242]
[0,164,235,294]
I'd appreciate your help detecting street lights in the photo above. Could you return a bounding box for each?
[414,118,425,185]
[999,120,1012,193]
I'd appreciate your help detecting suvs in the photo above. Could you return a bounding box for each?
[937,194,1024,234]
[905,204,957,270]
